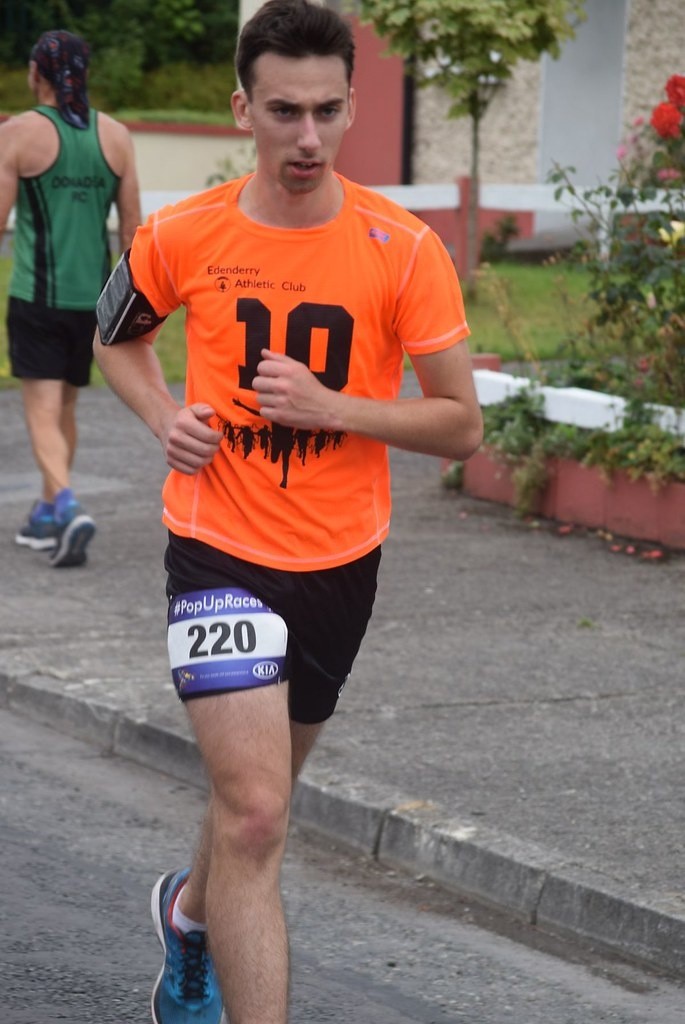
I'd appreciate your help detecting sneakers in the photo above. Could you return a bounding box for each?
[14,515,59,549]
[150,864,225,1024]
[47,506,95,567]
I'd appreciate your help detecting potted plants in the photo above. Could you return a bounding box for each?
[441,387,685,554]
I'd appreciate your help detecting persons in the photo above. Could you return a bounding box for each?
[94,0,482,1024]
[0,30,144,566]
